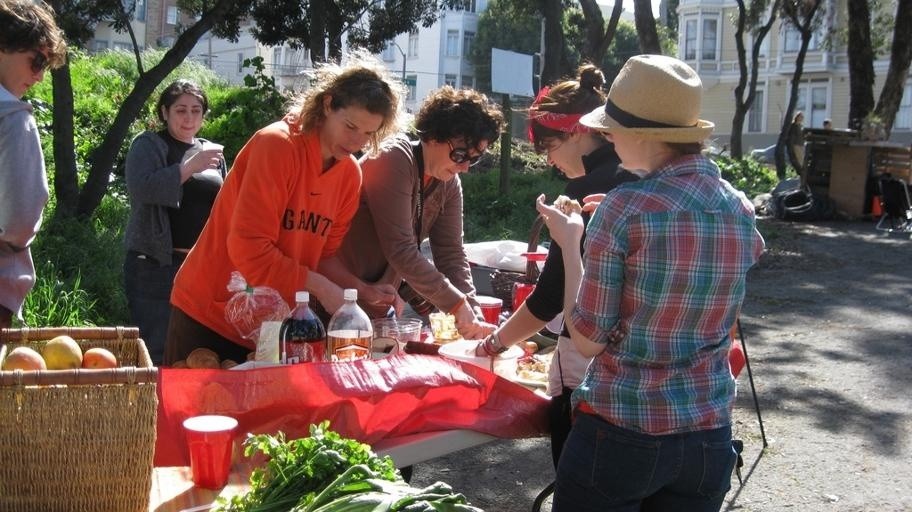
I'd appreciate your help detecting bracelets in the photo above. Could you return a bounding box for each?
[483,331,508,357]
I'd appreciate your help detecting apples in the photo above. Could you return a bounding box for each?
[43,336,83,370]
[2,345,47,371]
[83,348,117,370]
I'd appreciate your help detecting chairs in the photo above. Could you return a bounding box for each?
[875,176,912,235]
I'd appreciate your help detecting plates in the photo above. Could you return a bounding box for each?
[520,253,547,262]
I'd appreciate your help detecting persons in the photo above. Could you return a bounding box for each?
[789,113,805,145]
[823,121,832,129]
[309,85,510,339]
[0,0,68,328]
[163,48,411,367]
[536,54,766,512]
[475,64,648,470]
[124,79,227,366]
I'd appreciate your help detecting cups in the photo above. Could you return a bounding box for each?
[475,295,503,326]
[371,316,423,356]
[427,313,458,341]
[182,415,239,490]
[203,141,224,170]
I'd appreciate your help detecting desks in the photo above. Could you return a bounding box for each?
[827,139,905,220]
[150,381,498,511]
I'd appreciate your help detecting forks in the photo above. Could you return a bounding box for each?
[437,340,527,362]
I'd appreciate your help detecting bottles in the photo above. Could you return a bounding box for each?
[279,291,327,364]
[325,288,373,362]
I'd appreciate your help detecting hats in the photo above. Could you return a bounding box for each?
[579,55,715,144]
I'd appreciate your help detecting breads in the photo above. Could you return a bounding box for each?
[554,194,583,216]
[169,348,255,370]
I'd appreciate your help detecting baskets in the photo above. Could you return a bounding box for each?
[488,214,559,341]
[1,326,159,512]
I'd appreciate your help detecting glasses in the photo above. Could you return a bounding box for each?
[31,51,47,73]
[447,139,482,167]
